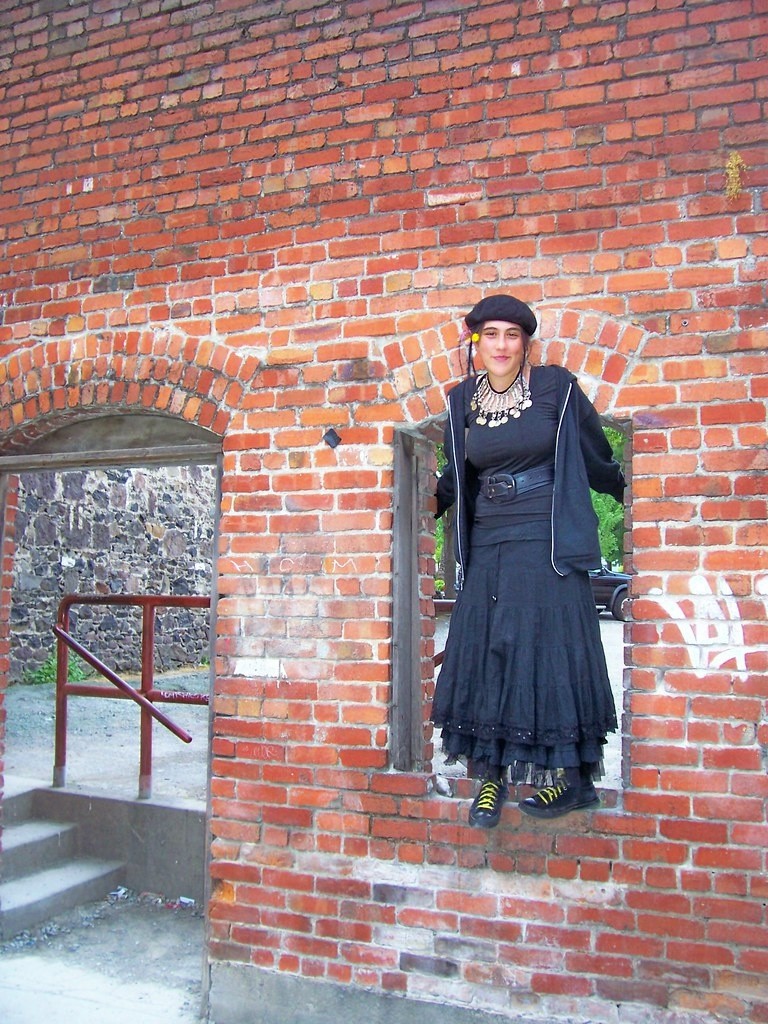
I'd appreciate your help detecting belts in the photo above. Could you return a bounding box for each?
[469,466,555,503]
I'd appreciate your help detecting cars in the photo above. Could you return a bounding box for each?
[587,556,632,622]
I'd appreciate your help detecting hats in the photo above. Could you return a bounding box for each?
[463,294,537,336]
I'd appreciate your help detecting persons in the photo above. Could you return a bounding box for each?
[436,295,624,829]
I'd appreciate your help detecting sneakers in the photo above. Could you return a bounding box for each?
[469,770,509,826]
[518,771,599,819]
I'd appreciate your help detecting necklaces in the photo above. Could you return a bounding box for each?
[471,368,532,428]
